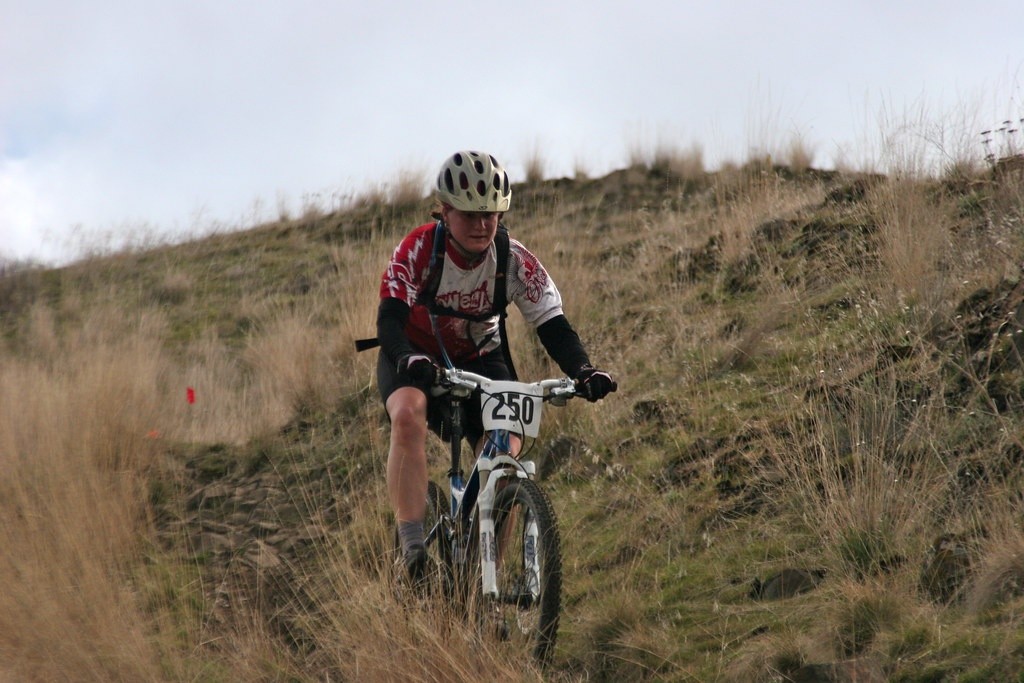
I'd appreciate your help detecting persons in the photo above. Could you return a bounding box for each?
[377,151,615,598]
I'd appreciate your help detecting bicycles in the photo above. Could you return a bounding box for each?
[384,359,617,676]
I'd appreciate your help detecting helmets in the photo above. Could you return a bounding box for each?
[435,151,512,212]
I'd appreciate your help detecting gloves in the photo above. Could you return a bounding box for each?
[576,367,615,402]
[408,354,436,389]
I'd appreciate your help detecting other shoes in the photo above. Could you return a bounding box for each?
[404,547,429,582]
